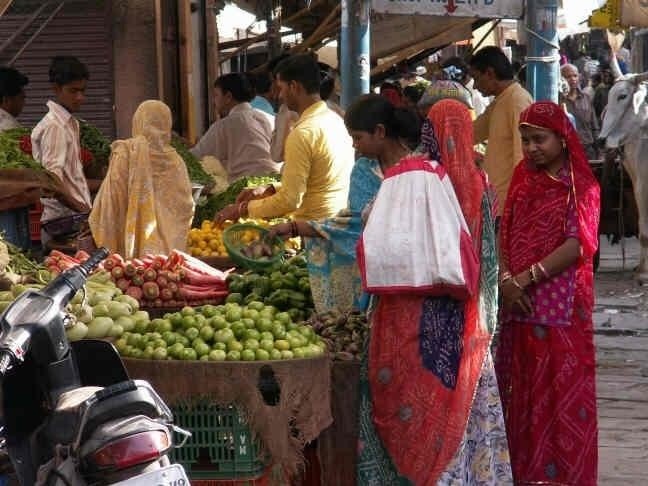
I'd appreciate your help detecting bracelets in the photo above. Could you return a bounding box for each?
[290,221,297,238]
[236,202,248,219]
[497,271,510,288]
[530,263,550,285]
[513,276,524,290]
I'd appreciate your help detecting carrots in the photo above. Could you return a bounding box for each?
[42,248,235,309]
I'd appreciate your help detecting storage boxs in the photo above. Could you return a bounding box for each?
[121,353,333,486]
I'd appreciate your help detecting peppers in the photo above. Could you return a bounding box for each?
[227,249,315,320]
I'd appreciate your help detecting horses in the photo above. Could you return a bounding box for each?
[599,71,648,286]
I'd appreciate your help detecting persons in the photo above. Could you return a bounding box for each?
[30,59,92,252]
[246,70,277,112]
[0,65,30,148]
[189,73,283,188]
[354,102,512,481]
[376,80,401,109]
[265,95,422,312]
[210,50,352,225]
[556,65,597,162]
[493,101,599,481]
[90,99,194,261]
[399,86,423,107]
[462,46,534,231]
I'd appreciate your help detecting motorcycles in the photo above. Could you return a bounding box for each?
[0,246,191,486]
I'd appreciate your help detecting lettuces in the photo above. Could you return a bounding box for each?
[0,127,44,171]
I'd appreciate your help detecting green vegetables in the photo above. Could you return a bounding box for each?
[310,310,368,360]
